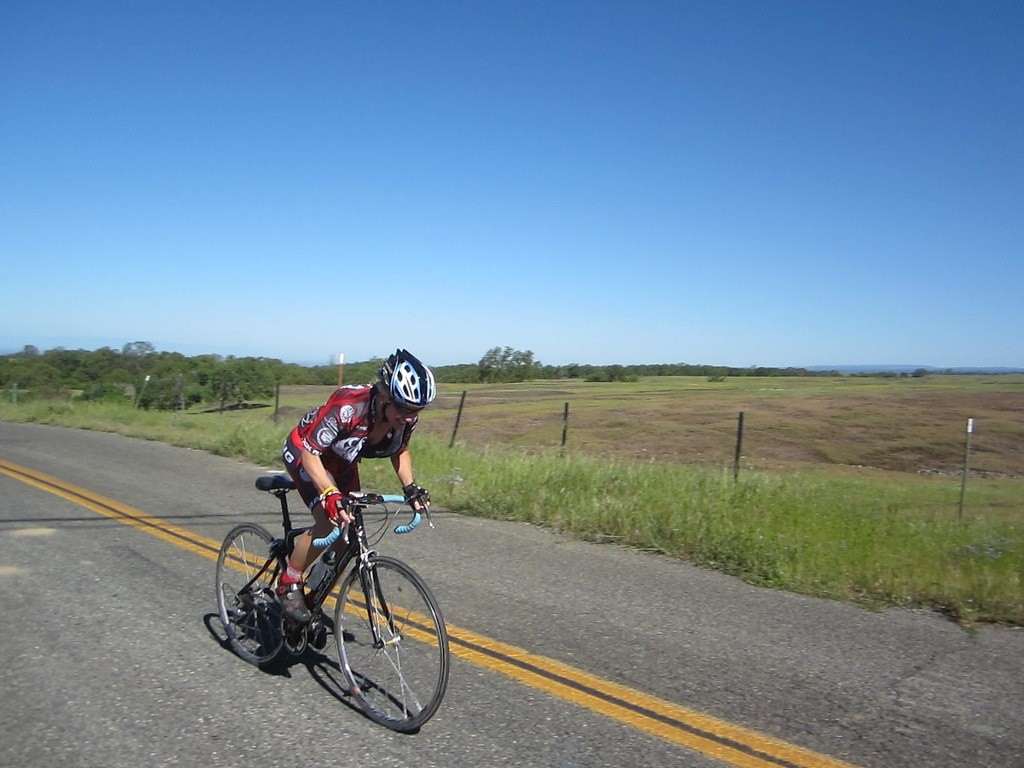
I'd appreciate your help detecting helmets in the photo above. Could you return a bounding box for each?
[378,348,437,409]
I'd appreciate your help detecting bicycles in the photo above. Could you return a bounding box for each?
[215,470,450,733]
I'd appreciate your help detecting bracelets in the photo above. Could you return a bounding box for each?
[320,486,339,496]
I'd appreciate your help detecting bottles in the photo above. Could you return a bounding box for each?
[307,551,336,589]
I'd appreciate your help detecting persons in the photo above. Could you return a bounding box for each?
[273,349,437,623]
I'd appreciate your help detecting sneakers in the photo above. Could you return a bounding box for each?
[272,573,311,622]
